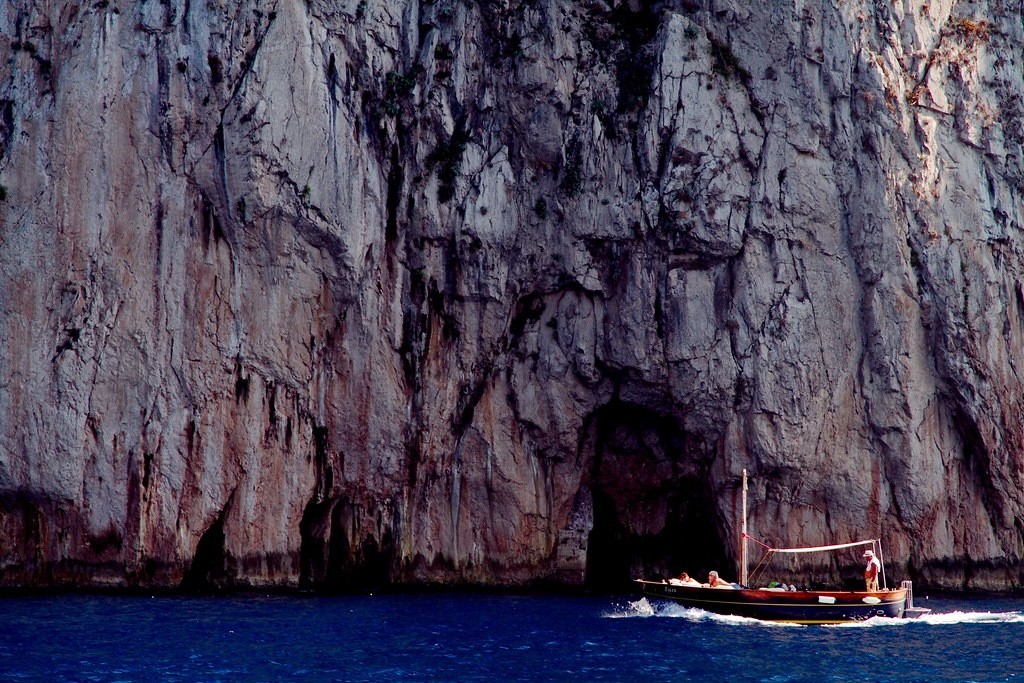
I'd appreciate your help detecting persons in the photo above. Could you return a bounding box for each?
[708,571,741,588]
[862,550,878,592]
[681,573,710,587]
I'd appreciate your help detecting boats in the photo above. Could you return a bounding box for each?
[632,468,931,624]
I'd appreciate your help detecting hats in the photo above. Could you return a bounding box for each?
[709,571,718,578]
[862,550,875,557]
[681,572,687,577]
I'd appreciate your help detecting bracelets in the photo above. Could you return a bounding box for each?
[871,582,873,583]
